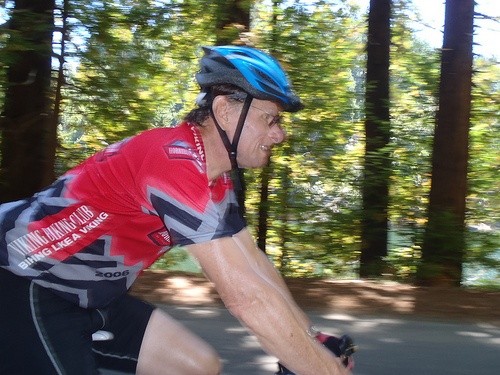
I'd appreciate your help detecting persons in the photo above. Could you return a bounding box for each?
[1,41,355,374]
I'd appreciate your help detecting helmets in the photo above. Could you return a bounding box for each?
[196,44,306,112]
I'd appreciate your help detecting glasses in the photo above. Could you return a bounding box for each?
[238,98,285,129]
